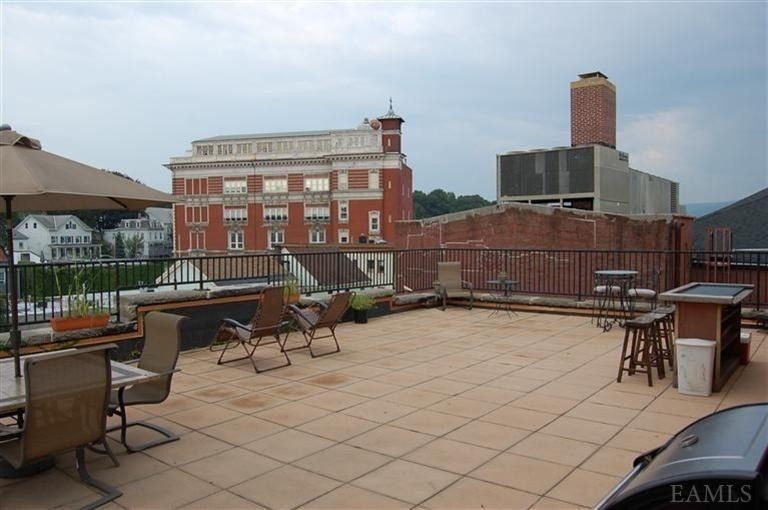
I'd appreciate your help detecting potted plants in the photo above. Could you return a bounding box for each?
[282,284,299,305]
[51,262,110,332]
[349,294,377,324]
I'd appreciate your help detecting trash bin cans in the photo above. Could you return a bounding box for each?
[674,338,717,397]
[740,332,751,366]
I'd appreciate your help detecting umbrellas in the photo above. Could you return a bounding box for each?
[0,123,193,445]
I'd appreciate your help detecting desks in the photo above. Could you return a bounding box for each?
[485,281,520,321]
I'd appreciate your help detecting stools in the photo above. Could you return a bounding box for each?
[592,285,676,387]
[595,270,638,333]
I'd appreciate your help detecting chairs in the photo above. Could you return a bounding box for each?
[209,284,357,373]
[1,311,189,509]
[433,261,474,311]
